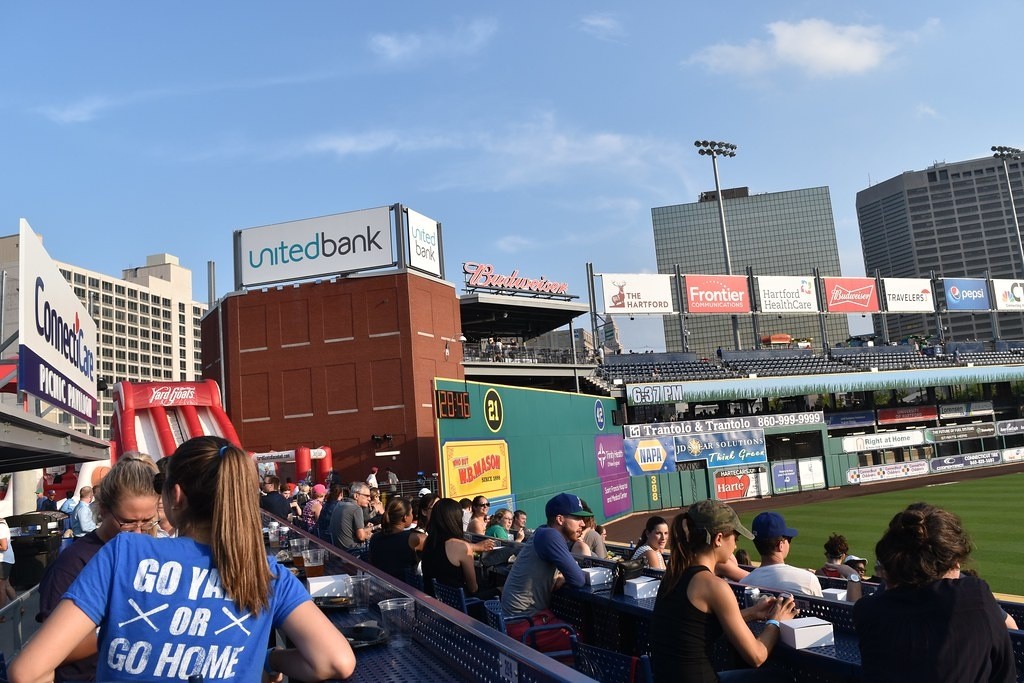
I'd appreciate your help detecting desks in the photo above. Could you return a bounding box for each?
[604,544,1024,630]
[464,532,1024,683]
[260,506,600,683]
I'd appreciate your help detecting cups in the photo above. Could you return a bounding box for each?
[463,533,472,542]
[750,593,774,624]
[301,549,326,578]
[342,575,371,615]
[493,540,501,547]
[377,597,415,648]
[289,537,310,566]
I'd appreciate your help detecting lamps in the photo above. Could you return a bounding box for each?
[444,332,467,362]
[862,313,866,317]
[1004,312,1008,316]
[972,312,975,315]
[314,279,322,285]
[732,317,736,320]
[293,283,300,289]
[825,316,827,318]
[503,311,508,319]
[899,313,904,318]
[97,377,108,392]
[276,285,284,291]
[261,287,269,293]
[329,278,337,284]
[628,313,634,320]
[778,313,782,319]
[383,434,393,448]
[372,434,383,448]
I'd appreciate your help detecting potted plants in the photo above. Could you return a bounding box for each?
[791,337,813,349]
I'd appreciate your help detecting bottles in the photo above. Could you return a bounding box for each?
[268,522,289,548]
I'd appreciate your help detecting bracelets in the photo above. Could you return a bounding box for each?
[766,619,779,627]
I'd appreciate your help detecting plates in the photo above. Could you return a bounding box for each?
[277,559,293,566]
[337,626,391,651]
[285,565,304,580]
[311,596,357,615]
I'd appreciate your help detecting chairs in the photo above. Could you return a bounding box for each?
[462,338,1024,389]
[0,529,654,683]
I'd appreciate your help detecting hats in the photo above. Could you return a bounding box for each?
[545,493,594,518]
[312,484,328,495]
[687,499,755,544]
[372,467,378,474]
[419,488,431,497]
[35,489,44,493]
[845,555,868,565]
[49,490,56,495]
[752,512,799,538]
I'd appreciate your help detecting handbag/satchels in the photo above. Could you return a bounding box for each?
[611,556,648,596]
[476,546,521,590]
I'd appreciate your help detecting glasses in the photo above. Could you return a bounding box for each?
[263,482,272,486]
[476,502,491,507]
[153,473,183,495]
[775,537,792,547]
[104,501,161,532]
[355,492,371,498]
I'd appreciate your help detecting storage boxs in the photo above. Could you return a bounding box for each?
[878,451,896,464]
[581,566,613,586]
[859,454,873,466]
[779,617,836,651]
[903,448,920,461]
[307,574,354,598]
[822,588,847,601]
[919,446,934,459]
[624,575,662,599]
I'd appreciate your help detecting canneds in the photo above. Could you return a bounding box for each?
[279,526,290,549]
[744,586,760,609]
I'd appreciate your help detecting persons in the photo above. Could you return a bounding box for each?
[654,498,800,683]
[856,503,1019,683]
[717,346,723,359]
[736,549,752,565]
[508,338,519,359]
[157,495,178,537]
[582,346,604,364]
[257,468,530,599]
[953,347,960,363]
[959,566,1018,630]
[494,338,504,361]
[36,450,162,683]
[8,435,357,683]
[485,338,495,352]
[630,515,751,582]
[738,511,823,597]
[815,532,861,579]
[696,406,762,419]
[0,518,25,621]
[34,486,102,542]
[502,492,609,649]
[843,555,868,577]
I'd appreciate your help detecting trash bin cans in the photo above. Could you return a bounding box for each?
[2,511,69,591]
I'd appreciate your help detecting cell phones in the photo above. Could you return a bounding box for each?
[768,592,793,614]
